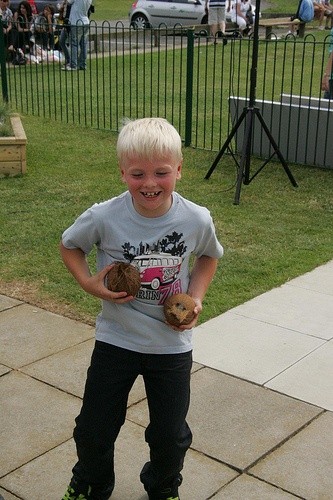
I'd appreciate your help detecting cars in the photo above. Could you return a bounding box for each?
[128,0,262,35]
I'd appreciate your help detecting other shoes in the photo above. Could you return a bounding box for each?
[224,39,227,45]
[62,66,77,71]
[212,41,218,45]
[78,65,86,69]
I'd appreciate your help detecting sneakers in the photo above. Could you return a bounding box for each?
[60,486,87,500]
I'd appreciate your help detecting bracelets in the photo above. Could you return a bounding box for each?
[323,72,331,78]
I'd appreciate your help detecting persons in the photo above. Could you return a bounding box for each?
[0,0,92,72]
[59,117,224,500]
[204,0,231,46]
[231,0,253,38]
[287,0,333,34]
[321,29,333,100]
